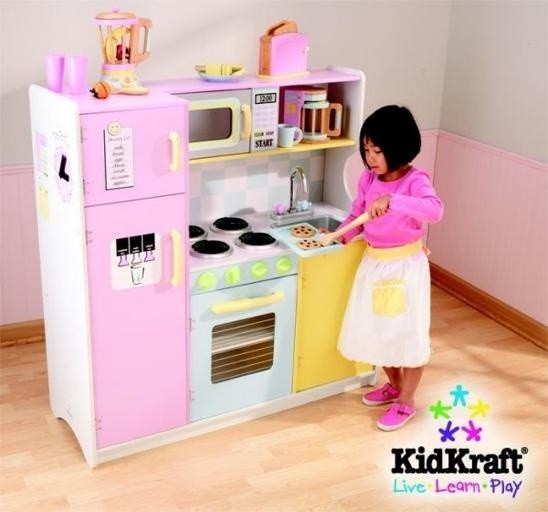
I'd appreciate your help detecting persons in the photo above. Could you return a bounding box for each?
[315,105,442,433]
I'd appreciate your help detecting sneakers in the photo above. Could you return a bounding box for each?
[361,383,400,406]
[376,403,417,431]
